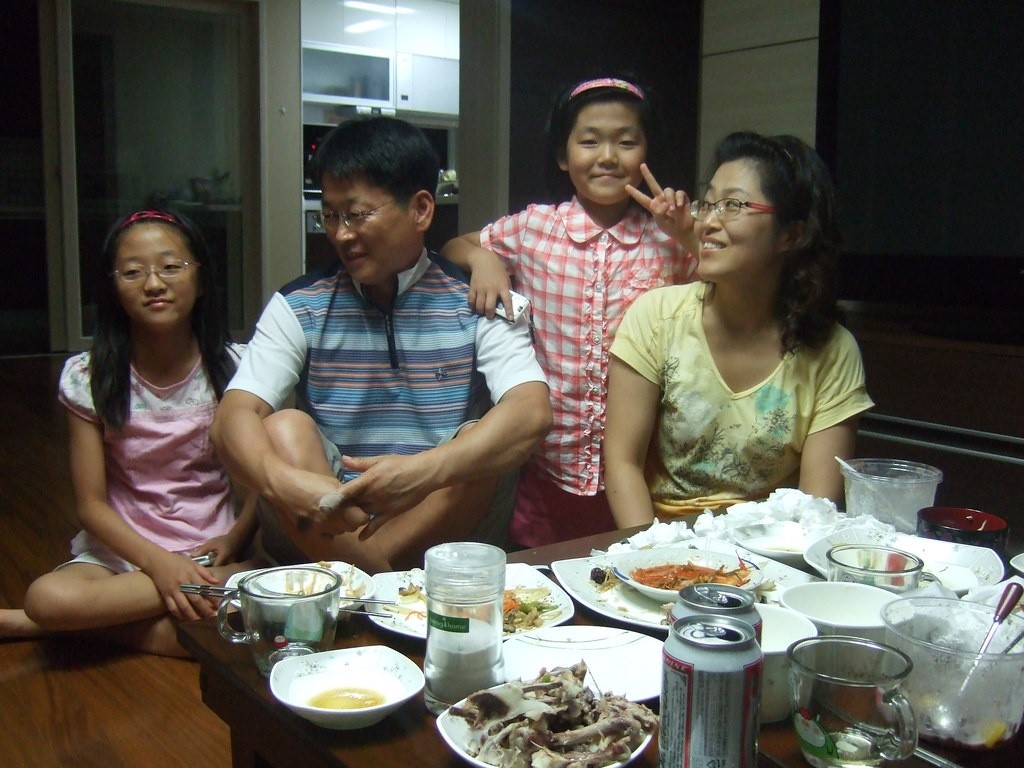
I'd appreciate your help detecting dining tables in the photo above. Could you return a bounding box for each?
[175,497,1024,768]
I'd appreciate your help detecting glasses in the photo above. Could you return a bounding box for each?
[109,259,201,283]
[320,199,395,227]
[690,197,777,221]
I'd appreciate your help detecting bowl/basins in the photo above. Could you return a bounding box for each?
[224,561,376,624]
[269,644,425,728]
[731,521,809,571]
[880,597,1024,751]
[611,547,764,603]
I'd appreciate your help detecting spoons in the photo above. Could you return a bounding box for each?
[834,456,914,531]
[922,583,1022,735]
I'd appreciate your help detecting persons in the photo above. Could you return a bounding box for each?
[598,130,873,536]
[210,116,556,576]
[441,67,700,552]
[0,203,294,661]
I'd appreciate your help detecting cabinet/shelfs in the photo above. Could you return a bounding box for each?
[395,1,460,128]
[301,0,398,127]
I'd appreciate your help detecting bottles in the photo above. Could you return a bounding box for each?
[422,541,506,717]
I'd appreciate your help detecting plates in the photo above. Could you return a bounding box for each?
[1009,552,1024,575]
[436,683,654,768]
[551,537,825,631]
[364,563,574,639]
[804,530,1003,594]
[503,626,665,705]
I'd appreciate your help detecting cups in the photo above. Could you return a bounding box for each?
[841,458,942,535]
[915,507,1008,562]
[753,604,817,725]
[787,635,918,768]
[826,544,943,595]
[779,583,914,671]
[217,567,343,678]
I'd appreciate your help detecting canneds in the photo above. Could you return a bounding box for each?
[657,614,764,768]
[666,582,762,646]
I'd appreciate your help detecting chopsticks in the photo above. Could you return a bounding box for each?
[816,696,962,768]
[180,584,394,620]
